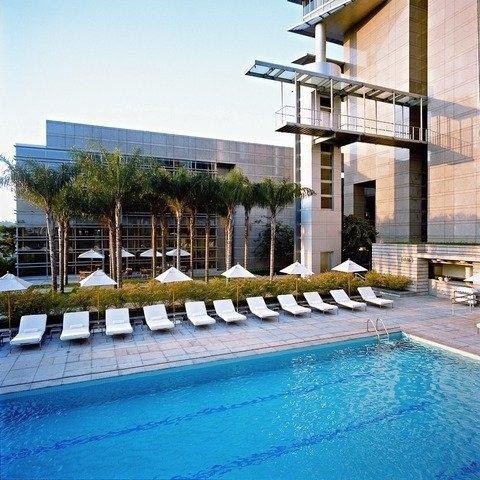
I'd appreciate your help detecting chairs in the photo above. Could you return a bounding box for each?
[11,313,47,349]
[143,304,175,333]
[213,299,247,325]
[78,265,188,280]
[330,289,366,311]
[277,293,312,317]
[303,291,338,314]
[357,286,393,309]
[186,300,216,329]
[245,296,279,321]
[60,312,90,343]
[105,308,133,339]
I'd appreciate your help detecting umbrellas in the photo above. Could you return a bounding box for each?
[279,261,314,300]
[166,249,192,257]
[78,269,120,329]
[108,247,135,269]
[463,271,480,283]
[77,248,105,272]
[153,267,193,322]
[219,262,256,314]
[330,258,368,299]
[0,270,33,327]
[139,249,164,258]
